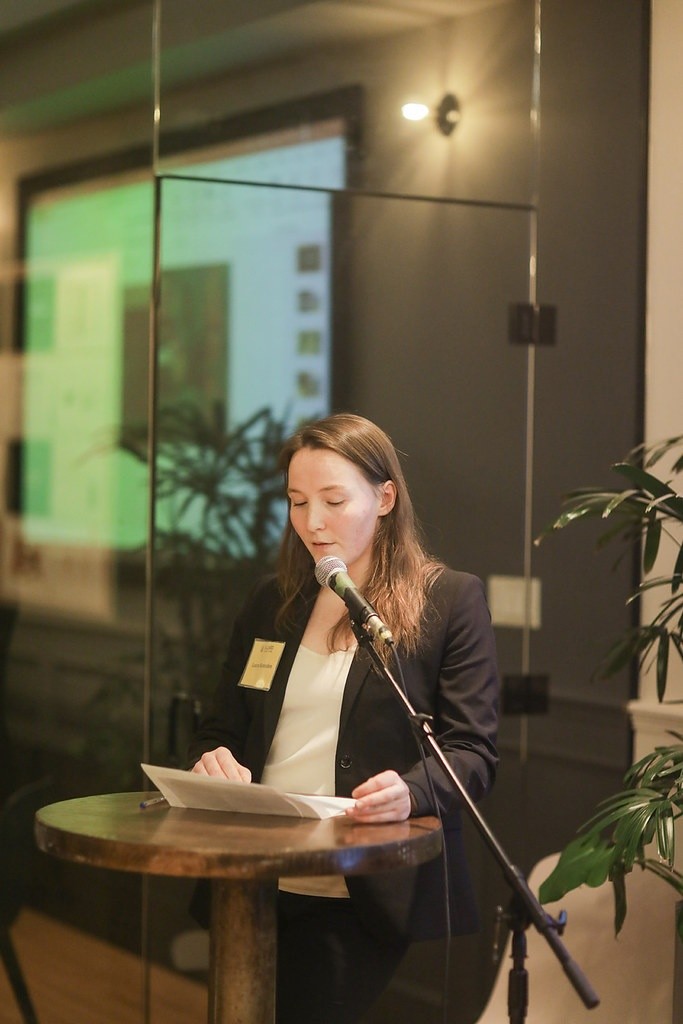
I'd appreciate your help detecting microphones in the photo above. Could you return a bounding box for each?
[314,556,394,646]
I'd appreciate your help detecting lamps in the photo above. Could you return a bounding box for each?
[402,95,461,127]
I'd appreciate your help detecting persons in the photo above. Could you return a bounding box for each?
[172,413,505,1024]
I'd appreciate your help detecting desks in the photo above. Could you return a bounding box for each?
[0,541,269,755]
[35,793,443,1024]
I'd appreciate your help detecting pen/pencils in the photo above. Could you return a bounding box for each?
[139,795,165,808]
